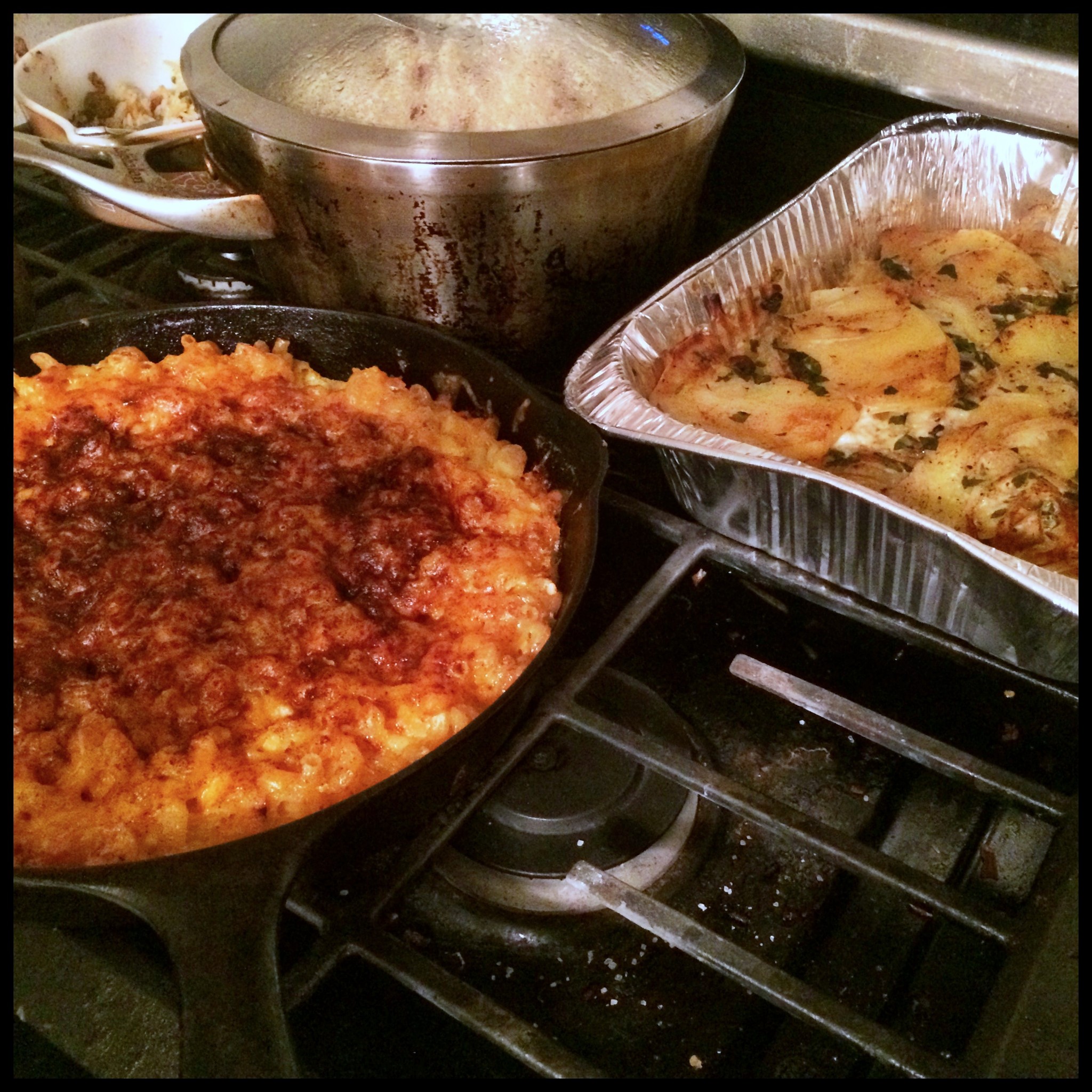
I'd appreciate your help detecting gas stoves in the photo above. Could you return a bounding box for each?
[11,8,1079,1083]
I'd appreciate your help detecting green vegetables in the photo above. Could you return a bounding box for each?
[722,254,1077,500]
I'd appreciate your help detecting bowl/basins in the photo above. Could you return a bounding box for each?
[566,109,1079,687]
[12,8,234,232]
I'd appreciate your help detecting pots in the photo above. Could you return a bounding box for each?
[10,9,748,358]
[12,295,613,1081]
